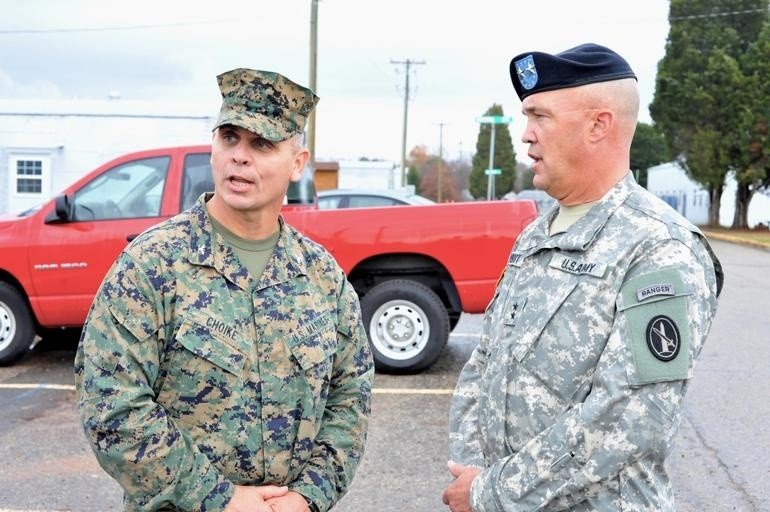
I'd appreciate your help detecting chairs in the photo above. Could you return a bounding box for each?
[189,180,216,204]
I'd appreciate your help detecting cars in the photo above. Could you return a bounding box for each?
[313,186,435,208]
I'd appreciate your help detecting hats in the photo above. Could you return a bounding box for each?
[509,44,638,102]
[211,67,320,143]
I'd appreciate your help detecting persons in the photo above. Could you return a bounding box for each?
[440,40,723,511]
[75,67,379,512]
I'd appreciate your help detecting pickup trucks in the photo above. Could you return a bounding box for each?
[0,143,539,371]
